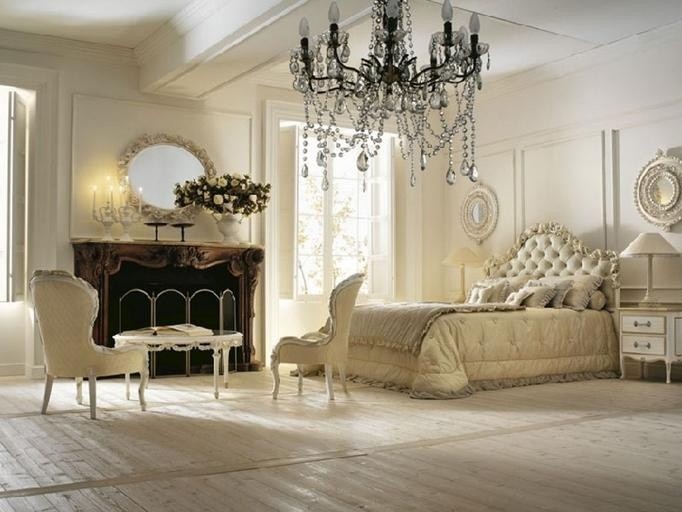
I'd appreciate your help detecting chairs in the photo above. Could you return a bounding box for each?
[29,269,150,419]
[271,273,365,399]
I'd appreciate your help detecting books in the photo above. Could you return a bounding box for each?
[122,322,215,337]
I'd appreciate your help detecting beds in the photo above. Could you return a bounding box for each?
[290,222,621,400]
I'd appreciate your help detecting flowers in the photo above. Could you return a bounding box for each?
[172,172,272,220]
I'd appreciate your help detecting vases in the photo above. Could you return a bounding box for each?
[217,203,239,243]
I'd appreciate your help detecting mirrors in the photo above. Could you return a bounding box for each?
[117,132,217,222]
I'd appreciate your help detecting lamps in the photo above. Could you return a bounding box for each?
[620,232,682,307]
[440,247,483,302]
[289,0,490,193]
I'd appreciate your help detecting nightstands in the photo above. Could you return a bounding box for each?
[614,307,682,384]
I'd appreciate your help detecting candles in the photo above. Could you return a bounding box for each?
[139,188,143,214]
[110,186,113,212]
[106,176,109,202]
[119,187,122,207]
[93,186,96,211]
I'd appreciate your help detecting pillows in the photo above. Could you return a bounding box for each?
[588,290,607,311]
[499,276,538,292]
[505,292,533,306]
[538,275,603,311]
[519,286,556,309]
[472,280,509,303]
[526,280,576,309]
[465,285,494,303]
